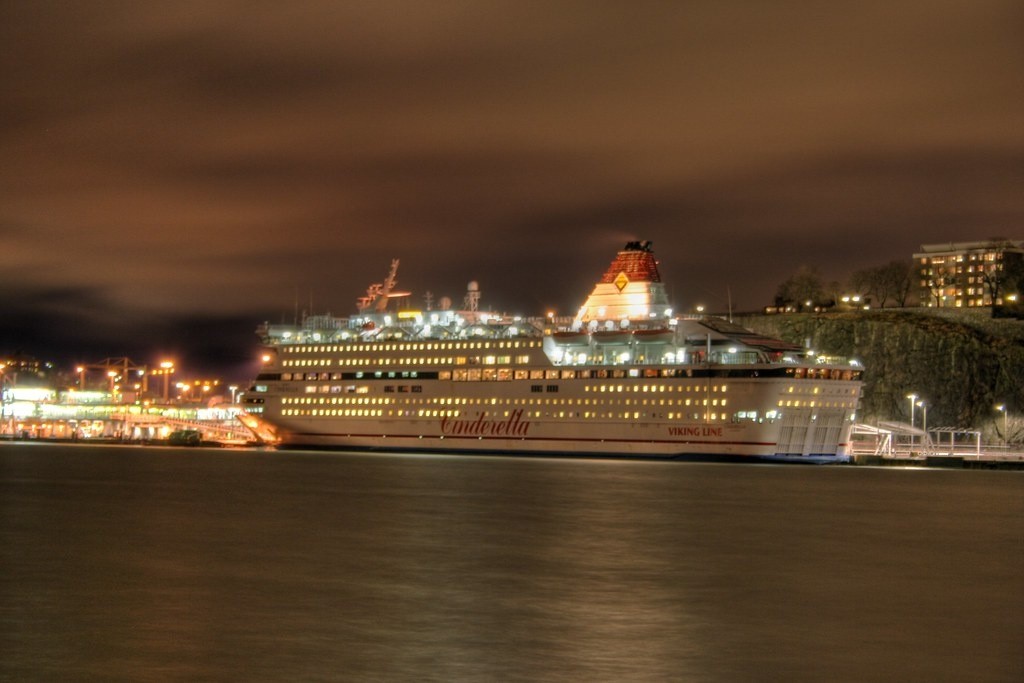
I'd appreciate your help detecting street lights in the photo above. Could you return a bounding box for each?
[997,404,1008,451]
[916,402,927,444]
[907,394,918,451]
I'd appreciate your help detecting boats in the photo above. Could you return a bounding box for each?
[232,305,866,464]
